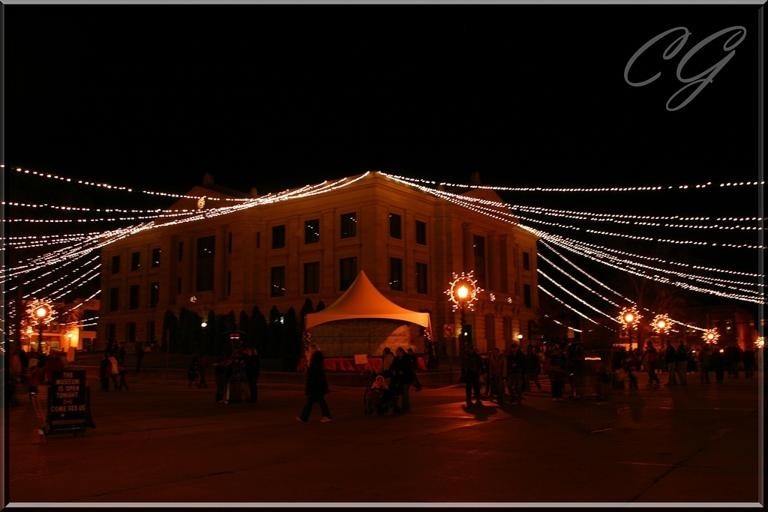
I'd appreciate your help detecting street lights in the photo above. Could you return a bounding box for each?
[648,313,674,351]
[443,270,485,325]
[615,307,643,354]
[26,298,59,355]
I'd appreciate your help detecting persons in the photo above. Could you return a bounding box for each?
[295,347,335,424]
[377,346,426,414]
[690,344,760,385]
[460,330,690,412]
[191,350,261,403]
[8,347,50,389]
[98,341,147,393]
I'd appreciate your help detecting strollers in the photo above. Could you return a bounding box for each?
[362,370,402,418]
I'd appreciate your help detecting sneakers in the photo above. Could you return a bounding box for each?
[321,417,332,422]
